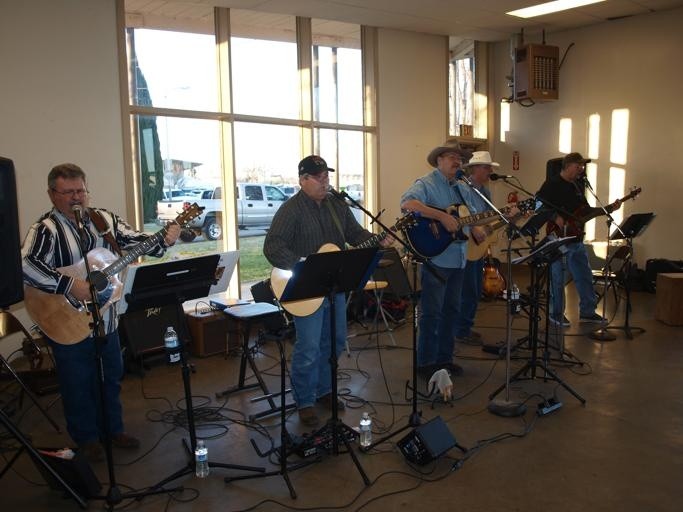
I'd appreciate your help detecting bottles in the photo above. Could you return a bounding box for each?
[512,284,520,311]
[360,413,371,446]
[195,440,209,479]
[165,328,181,363]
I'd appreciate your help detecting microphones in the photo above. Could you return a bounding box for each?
[581,171,590,189]
[455,170,472,184]
[71,205,82,228]
[327,184,344,200]
[489,172,515,182]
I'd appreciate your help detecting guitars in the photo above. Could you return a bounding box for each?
[20,202,207,347]
[482,243,507,300]
[469,206,534,262]
[400,197,537,260]
[268,209,419,318]
[547,185,646,242]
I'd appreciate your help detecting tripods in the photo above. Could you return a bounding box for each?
[224,324,325,499]
[500,229,571,358]
[79,230,183,507]
[136,286,265,501]
[597,238,646,340]
[279,285,372,487]
[488,250,587,407]
[367,259,469,456]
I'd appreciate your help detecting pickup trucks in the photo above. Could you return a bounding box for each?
[163,190,197,201]
[155,183,290,240]
[344,184,363,201]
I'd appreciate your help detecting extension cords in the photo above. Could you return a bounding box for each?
[537,398,562,414]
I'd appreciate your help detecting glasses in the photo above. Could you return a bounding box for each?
[571,165,585,170]
[52,189,91,198]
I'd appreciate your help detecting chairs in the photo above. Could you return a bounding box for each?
[344,268,396,357]
[0,312,64,433]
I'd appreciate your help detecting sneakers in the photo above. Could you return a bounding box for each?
[580,314,608,323]
[455,329,486,345]
[549,314,572,326]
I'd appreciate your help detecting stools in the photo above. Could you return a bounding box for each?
[217,302,300,423]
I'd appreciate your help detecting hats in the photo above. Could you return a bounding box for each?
[427,139,474,167]
[299,156,334,175]
[464,151,500,167]
[561,152,591,166]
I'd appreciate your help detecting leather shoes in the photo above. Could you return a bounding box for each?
[107,433,139,450]
[441,364,463,376]
[317,394,345,410]
[81,442,99,460]
[298,407,318,425]
[420,365,440,377]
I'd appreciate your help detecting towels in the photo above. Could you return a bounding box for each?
[428,368,452,402]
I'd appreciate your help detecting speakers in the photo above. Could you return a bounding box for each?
[515,44,561,101]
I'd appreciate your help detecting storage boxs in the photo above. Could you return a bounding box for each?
[657,272,683,327]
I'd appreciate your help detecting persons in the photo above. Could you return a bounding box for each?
[19,164,179,460]
[401,142,520,382]
[454,152,529,342]
[263,157,392,424]
[537,153,609,327]
